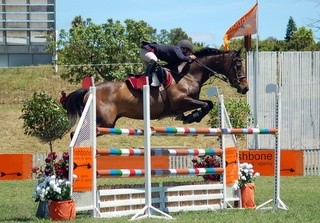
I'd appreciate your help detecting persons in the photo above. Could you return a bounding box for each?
[140,39,197,85]
[60,90,67,103]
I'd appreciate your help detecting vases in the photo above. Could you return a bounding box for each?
[233,183,256,208]
[36,198,50,220]
[48,199,77,222]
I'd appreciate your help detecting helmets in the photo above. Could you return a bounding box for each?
[177,40,194,53]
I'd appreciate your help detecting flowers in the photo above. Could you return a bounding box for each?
[33,152,78,201]
[192,154,228,180]
[237,165,261,189]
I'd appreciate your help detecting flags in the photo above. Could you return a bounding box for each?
[223,3,258,51]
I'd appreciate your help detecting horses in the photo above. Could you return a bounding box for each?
[57,43,250,148]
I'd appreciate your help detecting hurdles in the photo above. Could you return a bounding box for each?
[67,83,288,219]
